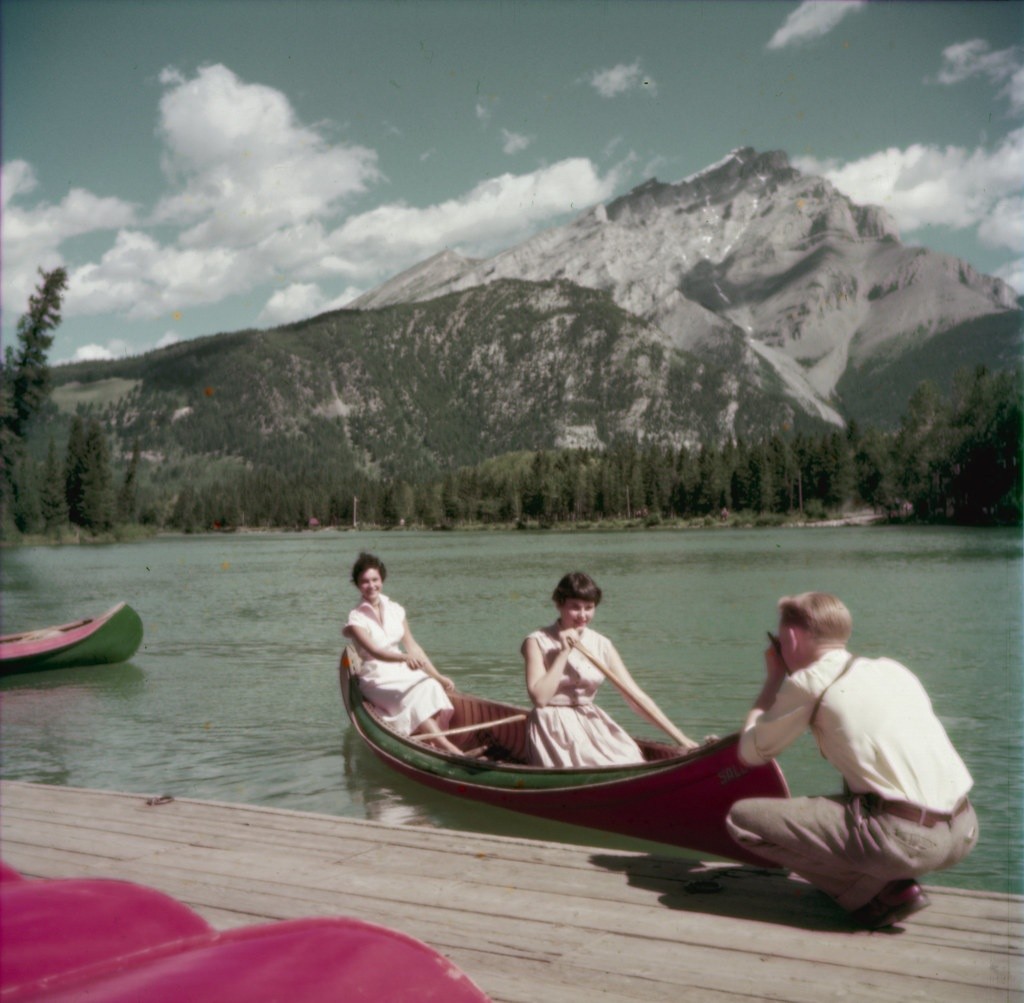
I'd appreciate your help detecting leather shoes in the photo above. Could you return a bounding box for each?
[846,879,931,930]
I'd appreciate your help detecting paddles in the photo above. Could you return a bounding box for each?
[420,662,451,688]
[568,635,698,748]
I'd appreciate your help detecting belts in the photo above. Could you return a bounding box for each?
[861,792,969,828]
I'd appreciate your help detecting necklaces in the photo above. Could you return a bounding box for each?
[371,601,379,606]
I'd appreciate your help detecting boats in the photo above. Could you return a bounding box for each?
[340,639,793,869]
[0,601,144,673]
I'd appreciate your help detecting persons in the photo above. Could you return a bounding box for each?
[342,551,465,755]
[725,591,980,932]
[522,571,682,768]
